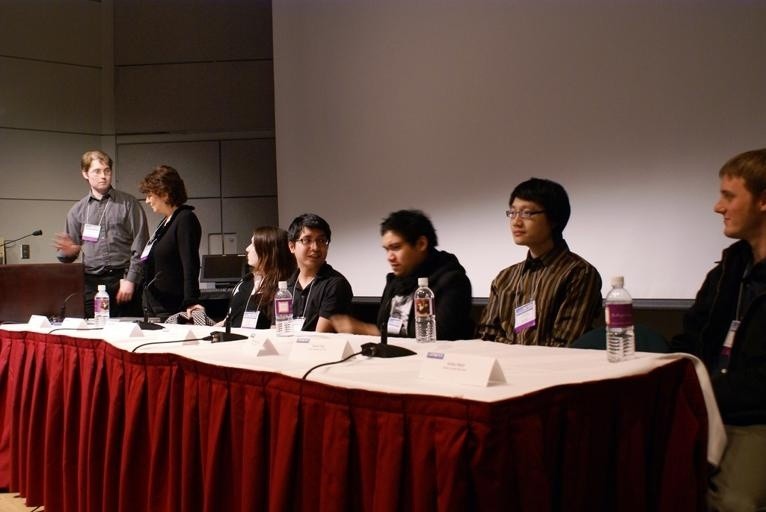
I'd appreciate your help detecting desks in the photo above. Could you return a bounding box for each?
[197,288,236,300]
[348,295,697,351]
[0,261,88,325]
[0,316,730,511]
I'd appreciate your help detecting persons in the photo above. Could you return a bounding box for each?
[283,213,352,333]
[666,149,765,512]
[330,209,477,339]
[50,149,149,318]
[222,227,297,329]
[475,177,603,349]
[138,166,205,319]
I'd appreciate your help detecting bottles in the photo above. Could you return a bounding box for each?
[271,279,294,337]
[93,284,110,326]
[412,276,440,344]
[604,276,638,363]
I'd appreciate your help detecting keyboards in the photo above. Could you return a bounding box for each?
[198,288,234,299]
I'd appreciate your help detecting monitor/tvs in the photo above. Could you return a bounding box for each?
[200,254,246,288]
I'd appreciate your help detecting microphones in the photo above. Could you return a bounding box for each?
[138,270,165,330]
[55,279,95,322]
[0,229,42,246]
[361,272,417,358]
[203,272,254,343]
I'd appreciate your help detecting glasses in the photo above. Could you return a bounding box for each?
[506,210,545,217]
[293,238,329,247]
